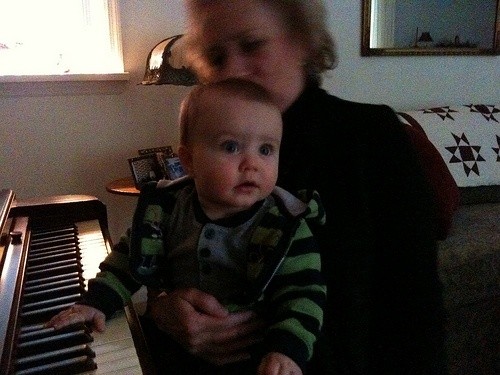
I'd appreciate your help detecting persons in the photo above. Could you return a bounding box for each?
[43,78,328,375]
[134,0,460,375]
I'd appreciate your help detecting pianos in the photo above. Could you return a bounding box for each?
[0,189,149,375]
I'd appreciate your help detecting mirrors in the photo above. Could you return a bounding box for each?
[360,0,500,56]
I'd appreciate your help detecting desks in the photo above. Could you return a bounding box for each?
[105,175,140,196]
